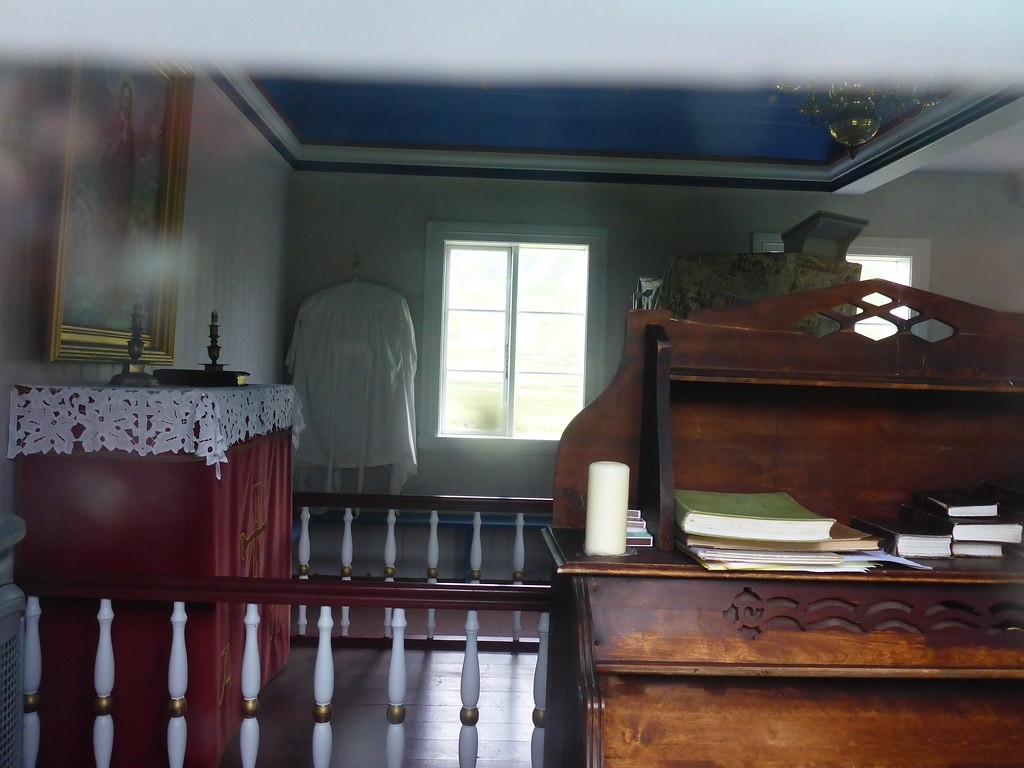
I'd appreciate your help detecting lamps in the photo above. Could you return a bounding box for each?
[776,77,942,159]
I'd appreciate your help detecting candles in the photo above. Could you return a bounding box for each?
[211,309,219,325]
[134,301,145,315]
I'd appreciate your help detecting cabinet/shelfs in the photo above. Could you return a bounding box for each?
[543,278,1024,768]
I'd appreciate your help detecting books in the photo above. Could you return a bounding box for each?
[849,517,952,559]
[674,539,934,574]
[154,368,250,387]
[674,522,880,551]
[899,504,1023,543]
[950,541,1004,557]
[913,491,1001,517]
[674,489,835,542]
[982,479,1024,557]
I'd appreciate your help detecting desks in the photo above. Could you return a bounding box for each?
[7,384,307,768]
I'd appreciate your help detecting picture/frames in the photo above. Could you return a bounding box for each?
[42,56,196,368]
